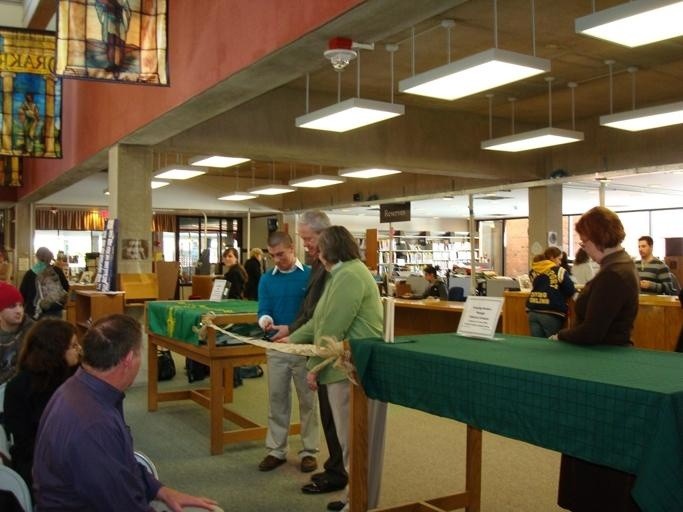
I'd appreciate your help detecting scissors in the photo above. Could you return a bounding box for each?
[249,321,275,341]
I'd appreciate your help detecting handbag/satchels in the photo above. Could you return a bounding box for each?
[158,351,176,380]
[186,340,210,381]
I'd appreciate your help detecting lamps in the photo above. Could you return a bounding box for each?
[575,0,683,48]
[155,156,206,181]
[249,161,296,195]
[190,154,250,168]
[481,76,584,152]
[295,44,405,134]
[599,62,683,132]
[399,1,550,101]
[339,167,401,179]
[289,166,344,188]
[217,167,258,201]
[150,181,168,189]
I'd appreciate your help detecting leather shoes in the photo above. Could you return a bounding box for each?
[302,473,336,494]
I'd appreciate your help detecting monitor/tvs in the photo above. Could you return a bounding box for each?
[267,218,279,231]
[380,270,390,295]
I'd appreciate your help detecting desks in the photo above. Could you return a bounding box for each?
[138,299,307,460]
[336,329,682,507]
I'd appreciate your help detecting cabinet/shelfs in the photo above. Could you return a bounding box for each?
[73,289,125,341]
[342,224,483,280]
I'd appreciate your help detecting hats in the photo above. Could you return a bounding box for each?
[36,247,56,261]
[0,280,24,310]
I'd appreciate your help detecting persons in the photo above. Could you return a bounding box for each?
[96,0,133,75]
[18,90,40,156]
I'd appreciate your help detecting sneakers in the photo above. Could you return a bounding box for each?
[259,455,286,470]
[327,501,345,511]
[301,456,317,472]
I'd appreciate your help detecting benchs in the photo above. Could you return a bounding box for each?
[0,383,157,512]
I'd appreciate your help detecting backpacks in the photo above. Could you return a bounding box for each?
[36,268,68,311]
